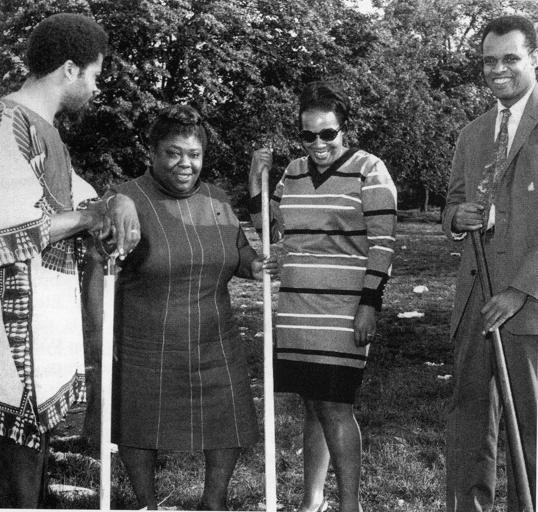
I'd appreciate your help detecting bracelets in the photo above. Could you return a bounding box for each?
[105,194,115,210]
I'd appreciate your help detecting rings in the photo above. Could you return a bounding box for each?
[367,333,373,337]
[131,228,139,234]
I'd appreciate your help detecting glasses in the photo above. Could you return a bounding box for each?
[297,125,345,143]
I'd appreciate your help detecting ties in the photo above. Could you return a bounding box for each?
[475,109,511,235]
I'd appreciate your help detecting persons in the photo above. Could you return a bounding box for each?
[440,13,538,512]
[0,12,144,509]
[249,78,400,511]
[93,101,281,510]
